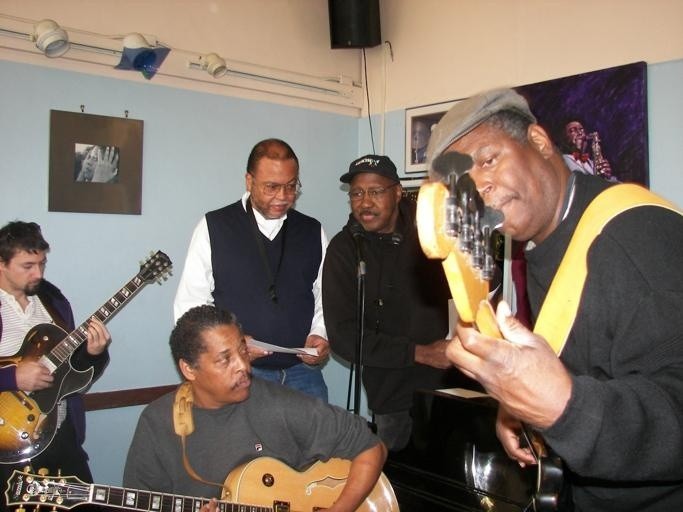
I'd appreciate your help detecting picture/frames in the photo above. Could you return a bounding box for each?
[404,98,472,174]
[48,110,143,216]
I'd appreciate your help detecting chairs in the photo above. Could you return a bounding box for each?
[386,459,525,510]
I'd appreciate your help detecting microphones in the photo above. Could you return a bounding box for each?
[349,221,367,279]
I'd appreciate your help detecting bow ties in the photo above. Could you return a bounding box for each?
[571,151,589,163]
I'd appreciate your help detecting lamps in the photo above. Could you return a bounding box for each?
[30,17,69,57]
[121,33,156,69]
[200,51,226,77]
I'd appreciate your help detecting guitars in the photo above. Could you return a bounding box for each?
[0,249,172,465]
[5,456,399,512]
[415,151,573,511]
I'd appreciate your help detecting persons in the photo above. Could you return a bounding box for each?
[560,120,618,183]
[172,139,331,405]
[321,154,456,451]
[0,221,112,485]
[122,305,389,512]
[426,90,683,511]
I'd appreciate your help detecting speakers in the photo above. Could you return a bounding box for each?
[328,0,381,49]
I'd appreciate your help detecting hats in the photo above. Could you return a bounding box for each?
[424,87,536,178]
[339,154,399,184]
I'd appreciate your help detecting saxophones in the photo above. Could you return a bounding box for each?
[581,132,605,178]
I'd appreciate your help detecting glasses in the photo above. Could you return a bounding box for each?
[248,175,301,196]
[347,181,400,199]
